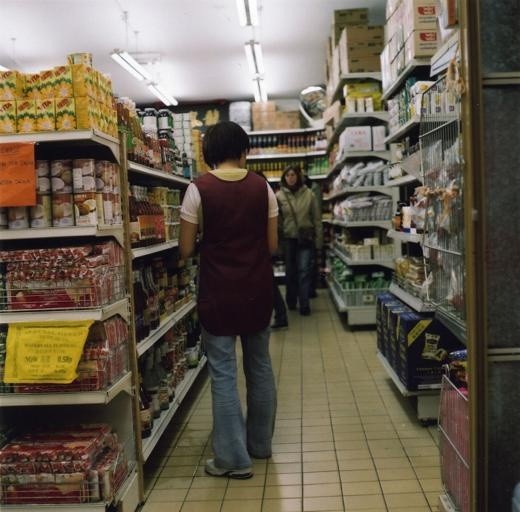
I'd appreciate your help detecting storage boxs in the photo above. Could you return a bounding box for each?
[230,0,449,128]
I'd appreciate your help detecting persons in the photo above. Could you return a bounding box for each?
[271,167,325,317]
[177,119,281,482]
[293,165,325,300]
[252,169,289,330]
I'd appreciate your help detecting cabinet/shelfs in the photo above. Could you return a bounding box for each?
[320,72,393,314]
[122,156,208,459]
[378,66,462,396]
[243,130,328,183]
[1,128,142,512]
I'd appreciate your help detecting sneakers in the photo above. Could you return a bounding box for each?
[205,457,253,480]
[249,449,273,459]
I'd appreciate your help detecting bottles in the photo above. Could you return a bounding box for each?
[115,94,194,178]
[125,181,182,247]
[138,311,203,439]
[244,131,330,179]
[130,265,161,341]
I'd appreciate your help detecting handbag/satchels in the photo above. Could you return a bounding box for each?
[297,225,316,250]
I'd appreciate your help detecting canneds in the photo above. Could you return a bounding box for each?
[136,107,175,147]
[0,158,123,229]
